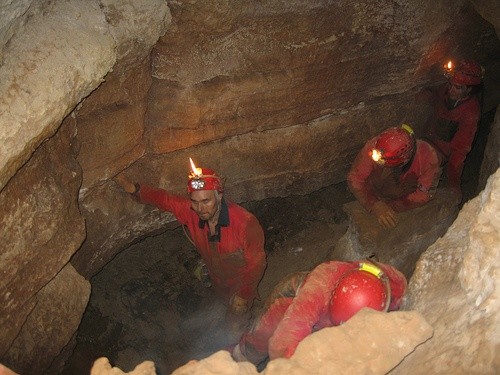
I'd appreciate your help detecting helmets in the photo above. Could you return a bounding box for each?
[328,262,390,326]
[444,56,482,85]
[376,127,414,168]
[188,168,220,192]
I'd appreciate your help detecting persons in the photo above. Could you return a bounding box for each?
[230,252,408,373]
[423,59,484,206]
[346,123,443,229]
[120,168,267,350]
